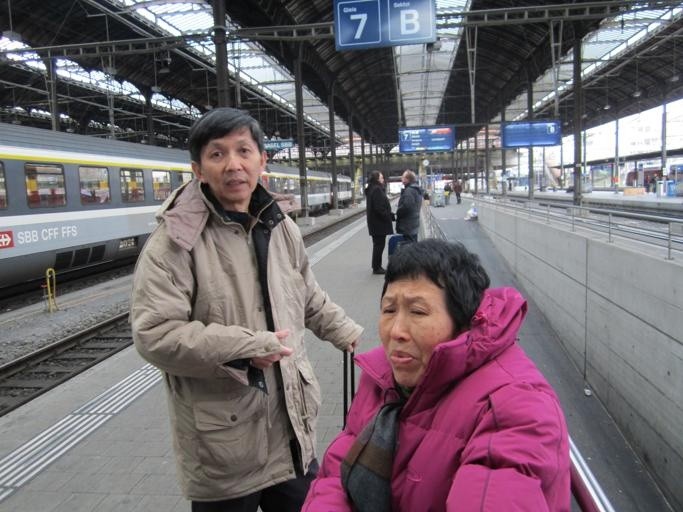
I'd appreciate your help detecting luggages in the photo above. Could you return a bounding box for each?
[388,235,415,256]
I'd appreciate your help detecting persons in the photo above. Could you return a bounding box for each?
[364,170,395,274]
[454,181,463,204]
[395,169,426,243]
[130,107,365,512]
[444,182,454,205]
[299,238,572,512]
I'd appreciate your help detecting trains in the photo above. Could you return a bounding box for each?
[0,120,357,294]
